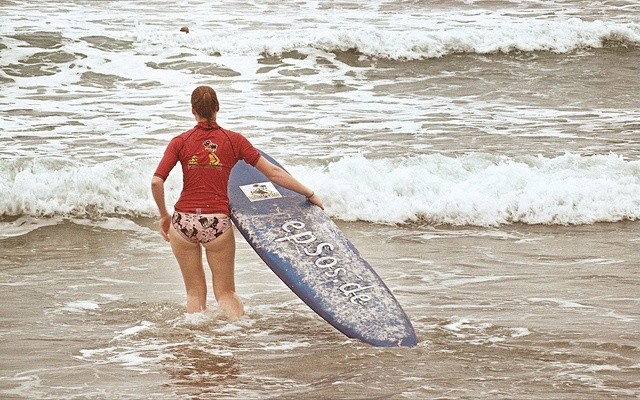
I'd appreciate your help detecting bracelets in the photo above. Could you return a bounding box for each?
[303,189,318,203]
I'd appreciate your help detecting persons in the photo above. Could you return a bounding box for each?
[148,83,323,321]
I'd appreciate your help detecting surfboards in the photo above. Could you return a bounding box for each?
[229,148,418,347]
